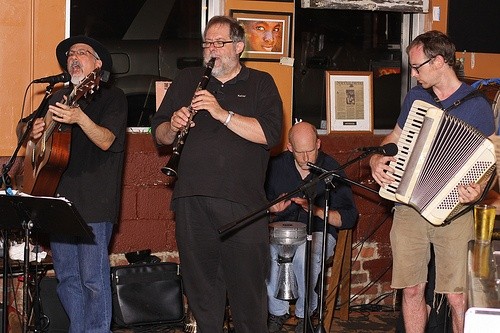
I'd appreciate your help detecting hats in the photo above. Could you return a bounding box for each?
[56,36,113,72]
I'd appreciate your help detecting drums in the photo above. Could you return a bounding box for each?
[270,222,308,301]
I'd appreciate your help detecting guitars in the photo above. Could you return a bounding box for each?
[21,67,106,197]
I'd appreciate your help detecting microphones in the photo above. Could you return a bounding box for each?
[32,73,71,84]
[355,143,398,156]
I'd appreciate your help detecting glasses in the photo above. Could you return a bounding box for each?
[201,40,234,48]
[408,58,432,74]
[65,49,99,60]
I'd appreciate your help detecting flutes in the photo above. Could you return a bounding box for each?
[160,57,216,177]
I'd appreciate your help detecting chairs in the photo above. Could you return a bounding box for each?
[288,226,353,332]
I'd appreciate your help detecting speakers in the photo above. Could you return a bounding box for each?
[28,274,71,333]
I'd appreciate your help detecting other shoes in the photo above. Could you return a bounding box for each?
[267,311,291,333]
[294,318,304,333]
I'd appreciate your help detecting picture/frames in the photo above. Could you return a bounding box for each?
[325,71,374,136]
[229,9,292,62]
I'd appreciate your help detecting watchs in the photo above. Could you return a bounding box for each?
[223,112,234,126]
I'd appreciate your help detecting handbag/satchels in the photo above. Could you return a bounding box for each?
[36,271,113,332]
[110,261,186,330]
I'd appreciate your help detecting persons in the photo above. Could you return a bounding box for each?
[346,96,354,103]
[16,36,131,333]
[240,20,282,52]
[370,29,499,333]
[152,16,283,333]
[265,122,359,333]
